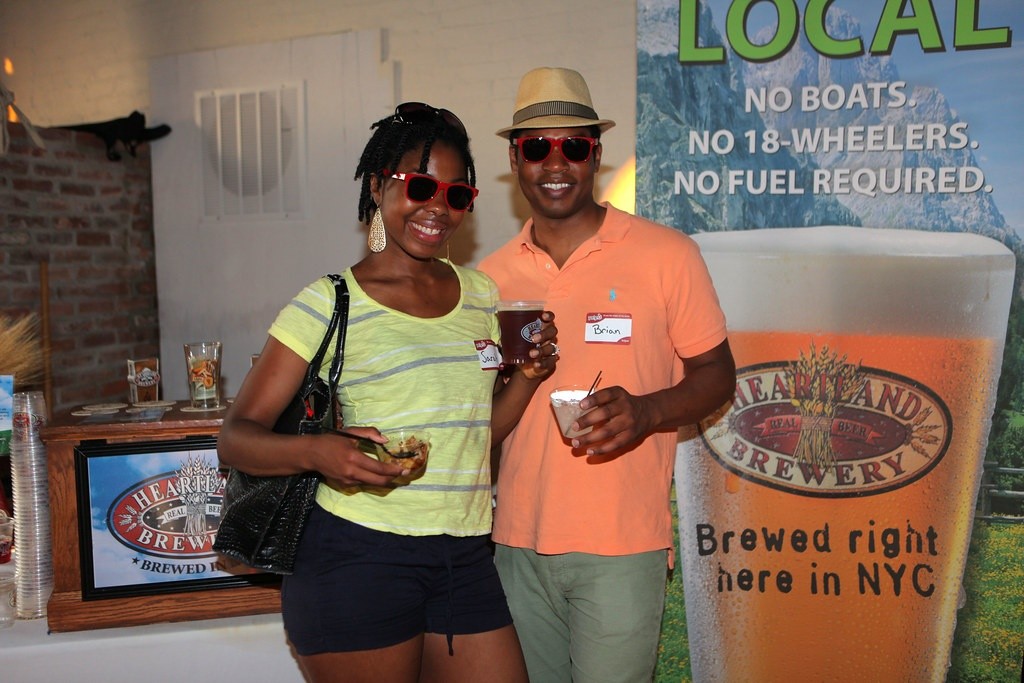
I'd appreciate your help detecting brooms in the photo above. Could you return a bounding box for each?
[0,309,50,389]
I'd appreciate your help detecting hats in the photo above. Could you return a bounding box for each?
[495,67,617,140]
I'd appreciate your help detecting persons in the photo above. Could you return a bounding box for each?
[216,104,561,683]
[476,67,736,683]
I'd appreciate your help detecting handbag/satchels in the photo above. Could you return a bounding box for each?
[212,273,349,576]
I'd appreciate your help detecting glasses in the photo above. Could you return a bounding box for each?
[394,102,469,145]
[510,136,599,163]
[381,169,480,213]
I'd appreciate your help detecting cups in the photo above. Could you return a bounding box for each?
[375,429,431,474]
[9,390,52,620]
[493,300,547,364]
[184,341,223,409]
[548,385,597,438]
[0,517,14,563]
[672,238,1016,683]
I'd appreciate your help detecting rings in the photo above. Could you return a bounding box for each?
[549,343,559,355]
